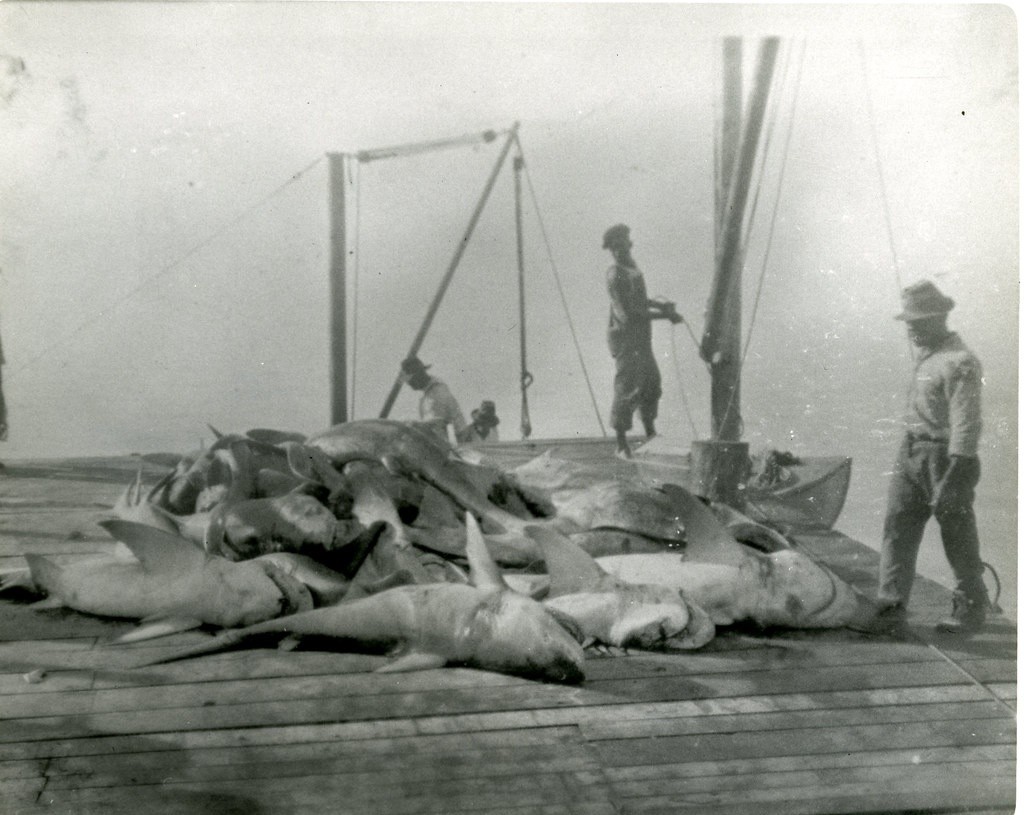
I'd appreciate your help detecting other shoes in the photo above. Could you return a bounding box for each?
[846,609,913,640]
[615,447,634,461]
[933,588,995,633]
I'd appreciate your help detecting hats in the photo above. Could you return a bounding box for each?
[398,355,434,383]
[894,280,955,324]
[470,399,500,428]
[602,224,633,249]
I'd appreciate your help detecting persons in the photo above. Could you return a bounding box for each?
[601,224,685,461]
[842,282,990,636]
[399,357,502,445]
